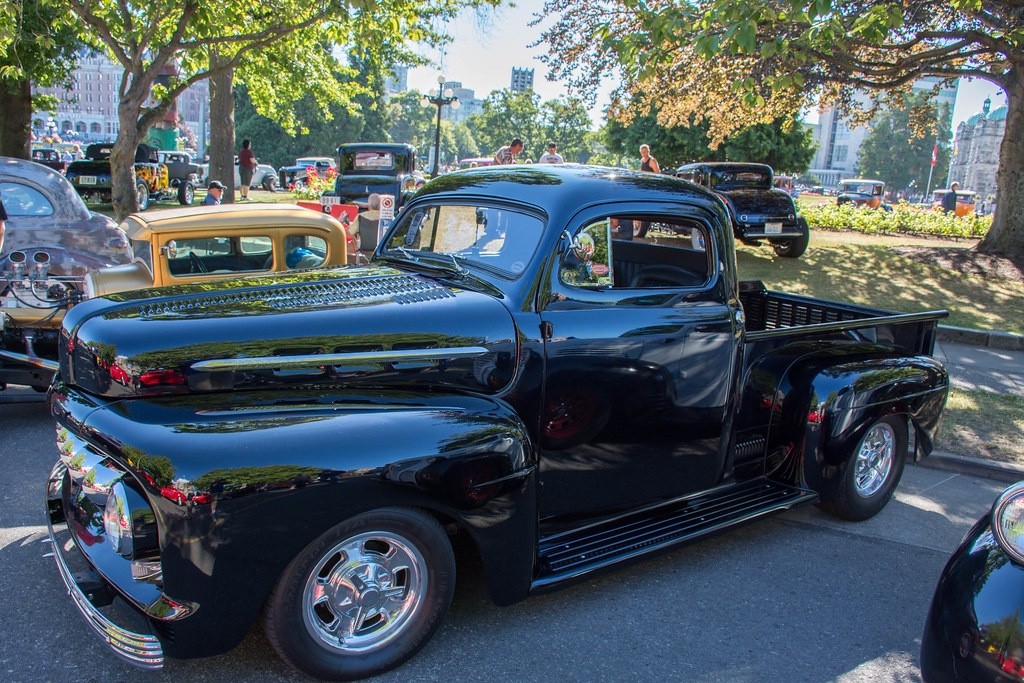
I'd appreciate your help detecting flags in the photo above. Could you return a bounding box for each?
[954,140,958,155]
[930,143,939,166]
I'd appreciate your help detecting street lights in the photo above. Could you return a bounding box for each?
[422,74,459,181]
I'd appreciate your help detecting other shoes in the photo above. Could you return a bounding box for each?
[241,196,253,201]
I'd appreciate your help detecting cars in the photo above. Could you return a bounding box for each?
[278,157,338,189]
[773,176,792,196]
[931,189,979,221]
[675,163,810,259]
[0,155,136,393]
[197,155,278,191]
[118,203,347,289]
[32,149,73,171]
[837,179,893,212]
[158,151,204,188]
[922,481,1023,683]
[321,143,427,218]
[794,184,839,197]
[67,144,195,212]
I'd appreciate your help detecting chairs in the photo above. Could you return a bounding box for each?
[629,265,708,287]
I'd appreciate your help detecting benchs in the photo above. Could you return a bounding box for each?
[263,245,325,270]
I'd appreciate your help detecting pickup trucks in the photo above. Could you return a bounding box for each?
[43,161,949,683]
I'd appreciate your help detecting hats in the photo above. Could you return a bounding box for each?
[549,143,556,148]
[207,180,227,189]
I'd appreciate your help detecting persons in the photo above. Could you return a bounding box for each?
[238,139,258,201]
[202,181,228,205]
[537,141,564,164]
[72,142,84,160]
[639,144,660,174]
[485,138,524,240]
[940,181,959,219]
